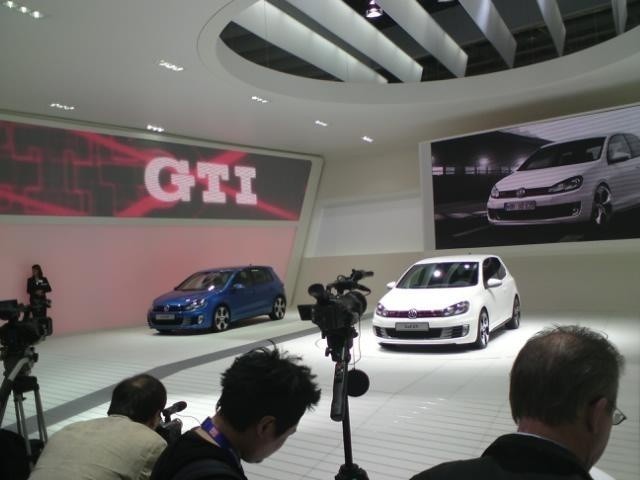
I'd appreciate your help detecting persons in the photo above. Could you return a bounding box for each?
[27,264,51,318]
[29,374,169,479]
[148,338,321,480]
[406,324,623,479]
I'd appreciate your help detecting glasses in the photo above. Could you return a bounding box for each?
[607,400,628,426]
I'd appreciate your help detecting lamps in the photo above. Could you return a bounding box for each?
[365,0,383,18]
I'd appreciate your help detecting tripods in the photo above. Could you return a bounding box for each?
[0,350,48,473]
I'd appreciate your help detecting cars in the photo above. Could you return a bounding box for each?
[487,132,639,227]
[371,254,520,348]
[147,265,287,333]
